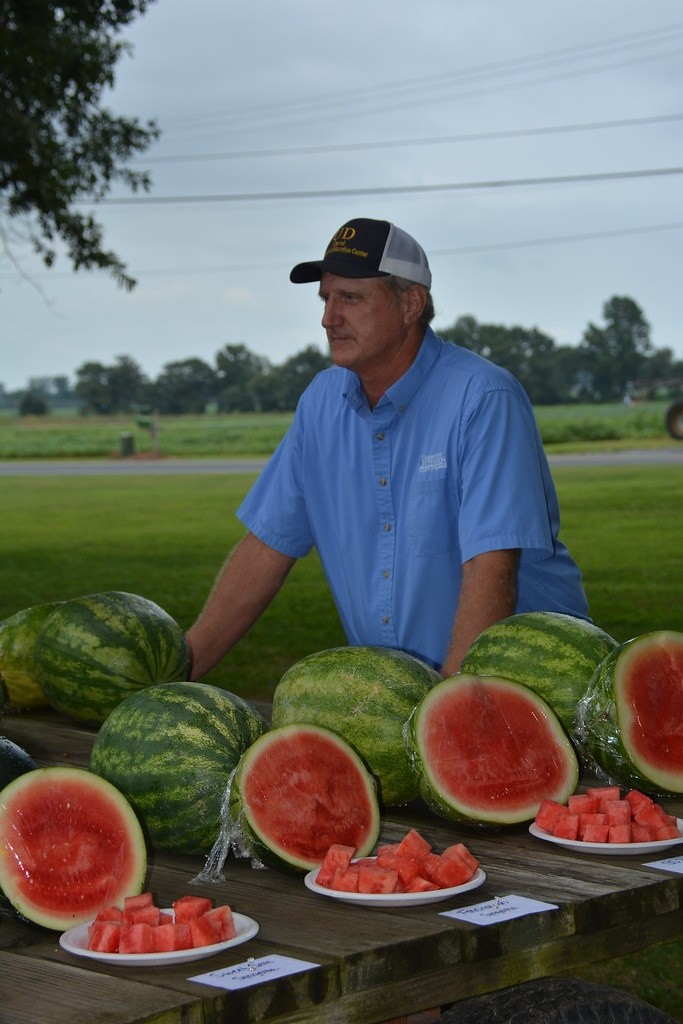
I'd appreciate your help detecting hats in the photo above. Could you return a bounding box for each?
[290,218,432,290]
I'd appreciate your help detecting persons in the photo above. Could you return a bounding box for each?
[183,218,592,683]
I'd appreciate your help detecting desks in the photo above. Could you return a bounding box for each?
[0,701,683,1024]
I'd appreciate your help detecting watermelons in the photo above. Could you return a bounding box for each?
[0,591,683,955]
